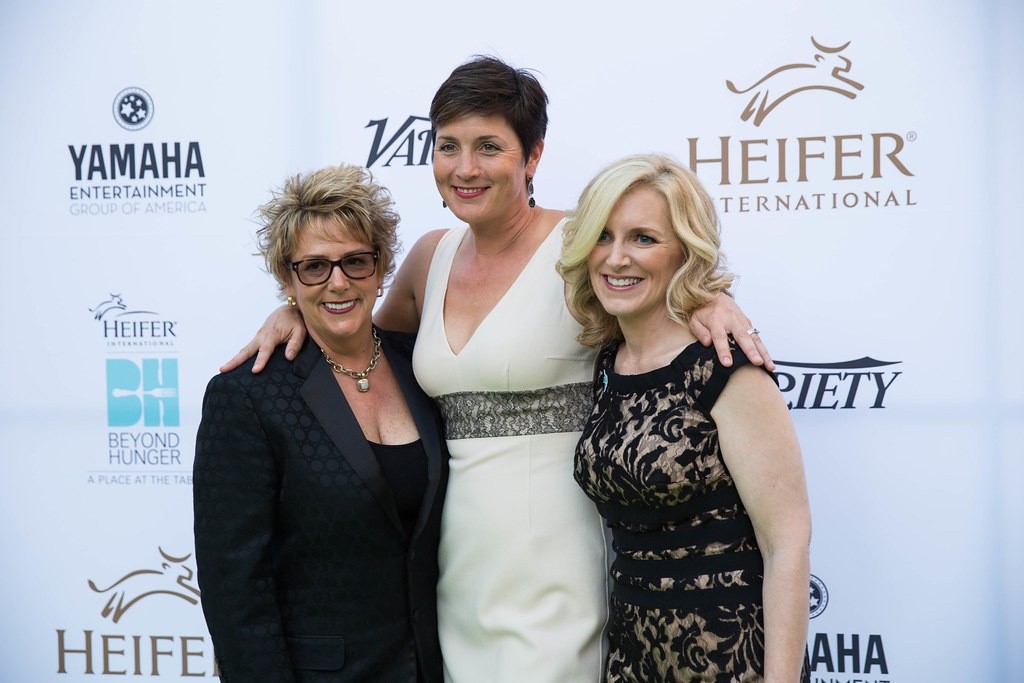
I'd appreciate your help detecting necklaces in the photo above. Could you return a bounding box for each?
[321,328,381,392]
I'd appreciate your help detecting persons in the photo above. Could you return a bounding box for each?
[219,54,775,683]
[555,152,812,683]
[192,164,449,683]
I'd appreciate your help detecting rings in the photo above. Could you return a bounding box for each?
[747,328,760,336]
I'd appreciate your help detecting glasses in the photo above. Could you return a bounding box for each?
[286,250,378,287]
